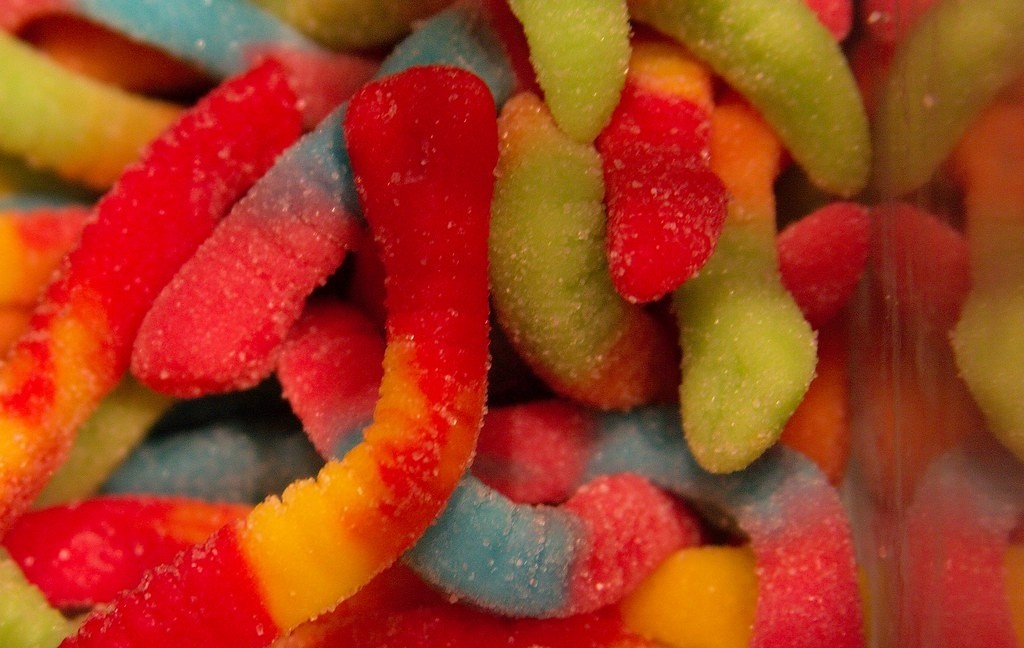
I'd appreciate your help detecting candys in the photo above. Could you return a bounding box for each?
[0,0,1024,648]
